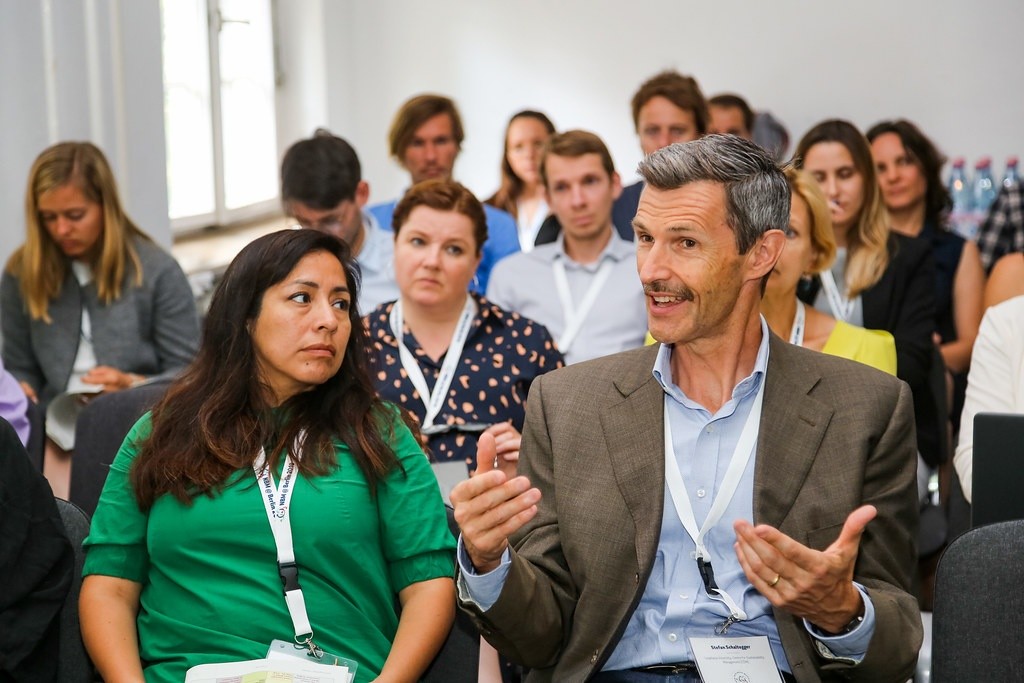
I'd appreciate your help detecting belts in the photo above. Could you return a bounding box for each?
[629,662,797,683]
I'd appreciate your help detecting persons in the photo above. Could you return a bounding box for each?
[0,142,204,502]
[360,178,567,683]
[76,229,458,683]
[448,133,926,683]
[280,70,1024,508]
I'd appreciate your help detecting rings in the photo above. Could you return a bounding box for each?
[769,574,780,588]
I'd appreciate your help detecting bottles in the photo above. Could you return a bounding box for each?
[947,156,1018,239]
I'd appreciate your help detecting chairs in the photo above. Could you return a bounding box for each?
[41,496,1023,683]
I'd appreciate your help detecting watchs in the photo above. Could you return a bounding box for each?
[840,610,864,637]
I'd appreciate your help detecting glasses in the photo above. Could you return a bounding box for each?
[285,202,349,233]
[419,423,493,435]
